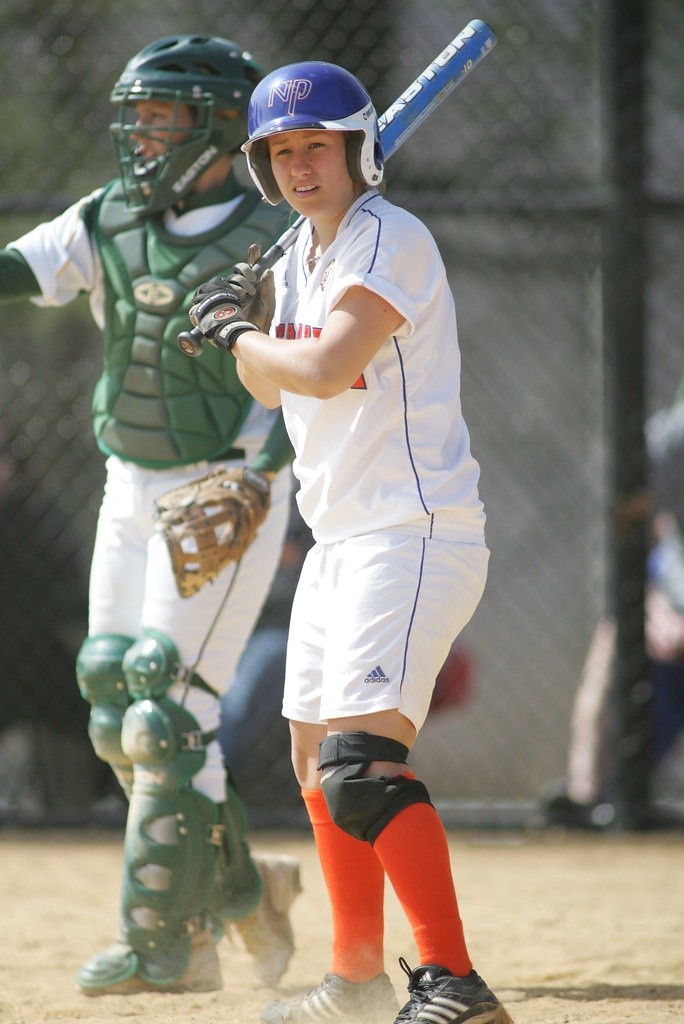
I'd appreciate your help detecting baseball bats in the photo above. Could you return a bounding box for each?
[174,19,501,357]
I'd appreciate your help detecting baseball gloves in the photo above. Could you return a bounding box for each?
[150,466,274,601]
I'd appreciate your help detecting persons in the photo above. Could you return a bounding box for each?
[0,35,302,993]
[188,61,509,1024]
[0,385,684,839]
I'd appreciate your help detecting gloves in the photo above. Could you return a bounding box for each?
[187,276,261,351]
[226,244,276,335]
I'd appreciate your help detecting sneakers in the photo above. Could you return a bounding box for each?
[260,971,400,1024]
[392,957,514,1024]
[77,929,222,996]
[223,856,306,988]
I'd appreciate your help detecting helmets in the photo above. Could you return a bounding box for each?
[240,60,384,207]
[108,33,266,215]
